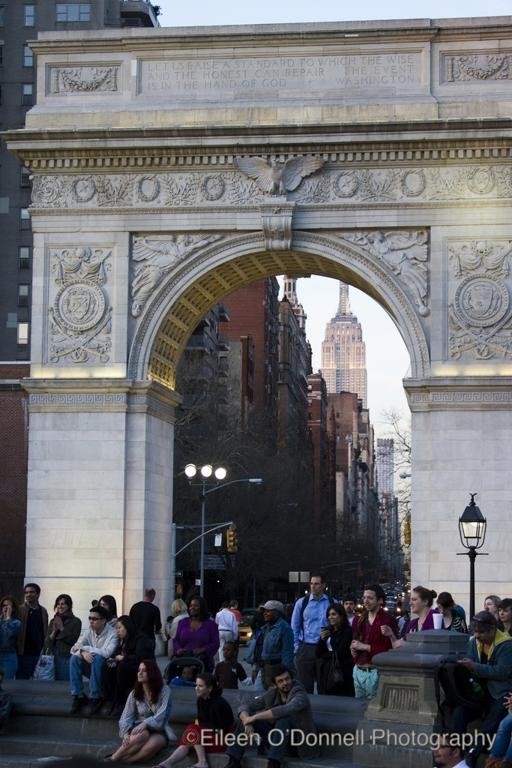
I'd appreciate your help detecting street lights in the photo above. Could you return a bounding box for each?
[179,459,230,606]
[200,476,264,599]
[453,490,493,622]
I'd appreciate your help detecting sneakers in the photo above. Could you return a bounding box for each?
[68,695,121,718]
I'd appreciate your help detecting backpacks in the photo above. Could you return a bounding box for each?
[434,655,473,722]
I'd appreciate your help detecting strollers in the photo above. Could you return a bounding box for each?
[162,646,207,687]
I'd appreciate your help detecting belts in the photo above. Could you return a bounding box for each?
[218,629,231,632]
[357,666,371,673]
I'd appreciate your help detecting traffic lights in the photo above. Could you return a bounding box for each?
[225,526,240,553]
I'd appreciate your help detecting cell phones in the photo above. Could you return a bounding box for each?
[321,627,328,631]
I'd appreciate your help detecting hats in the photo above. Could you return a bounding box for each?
[259,600,285,613]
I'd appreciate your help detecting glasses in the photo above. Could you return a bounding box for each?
[88,615,105,621]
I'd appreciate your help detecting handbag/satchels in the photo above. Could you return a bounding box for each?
[32,644,56,681]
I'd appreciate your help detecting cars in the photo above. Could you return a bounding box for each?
[232,577,412,644]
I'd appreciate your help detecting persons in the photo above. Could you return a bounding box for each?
[132,234,221,317]
[336,230,429,317]
